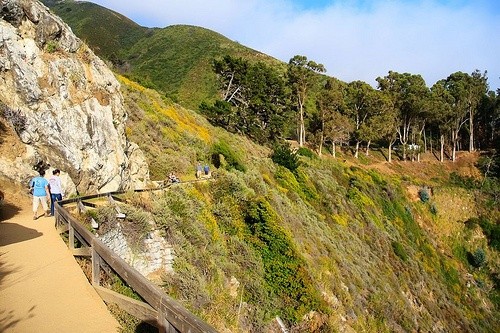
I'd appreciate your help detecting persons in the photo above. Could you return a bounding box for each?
[49,169,62,216]
[197,163,202,178]
[204,163,210,175]
[29,171,51,220]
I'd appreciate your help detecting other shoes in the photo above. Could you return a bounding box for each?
[33,216,37,220]
[45,213,51,217]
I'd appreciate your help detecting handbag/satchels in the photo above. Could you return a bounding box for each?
[26,186,33,198]
